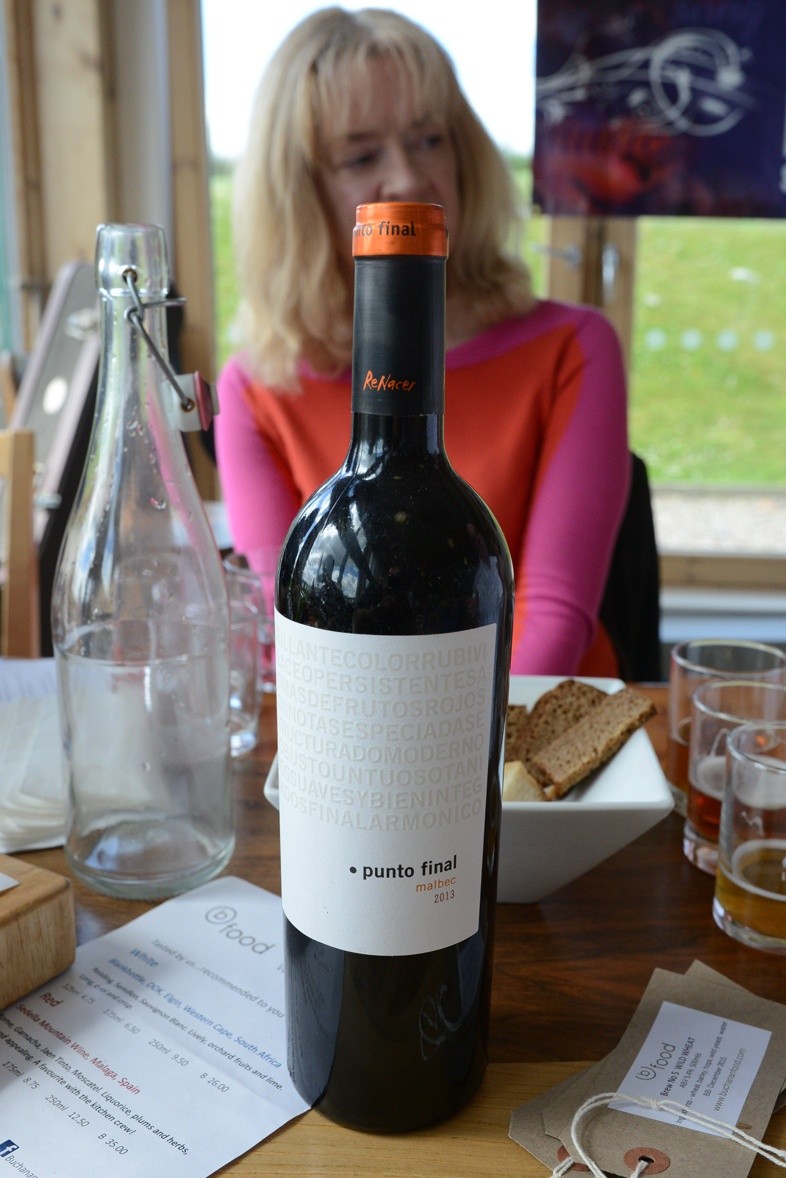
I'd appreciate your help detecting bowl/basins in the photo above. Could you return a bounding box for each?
[264,676,675,903]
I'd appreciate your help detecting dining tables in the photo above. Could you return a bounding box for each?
[0,685,786,1178]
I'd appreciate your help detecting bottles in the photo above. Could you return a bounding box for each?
[274,203,516,1135]
[51,224,235,899]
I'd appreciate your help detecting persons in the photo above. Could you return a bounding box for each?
[214,9,629,676]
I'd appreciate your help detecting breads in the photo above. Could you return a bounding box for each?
[505,680,658,801]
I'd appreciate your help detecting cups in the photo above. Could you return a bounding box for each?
[667,639,786,816]
[150,602,259,758]
[712,721,786,956]
[684,680,786,877]
[152,554,277,694]
